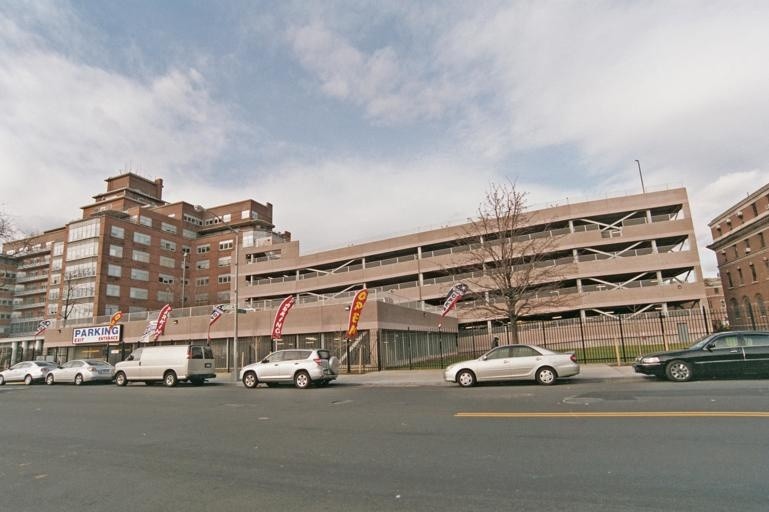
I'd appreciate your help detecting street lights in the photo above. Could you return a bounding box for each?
[194,205,240,382]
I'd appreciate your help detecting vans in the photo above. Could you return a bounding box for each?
[112,345,216,387]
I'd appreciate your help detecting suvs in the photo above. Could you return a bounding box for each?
[239,349,341,388]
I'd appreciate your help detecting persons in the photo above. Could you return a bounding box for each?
[490,337,499,349]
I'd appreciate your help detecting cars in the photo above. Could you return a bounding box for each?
[0,361,58,385]
[632,331,769,382]
[44,359,115,385]
[444,343,580,387]
